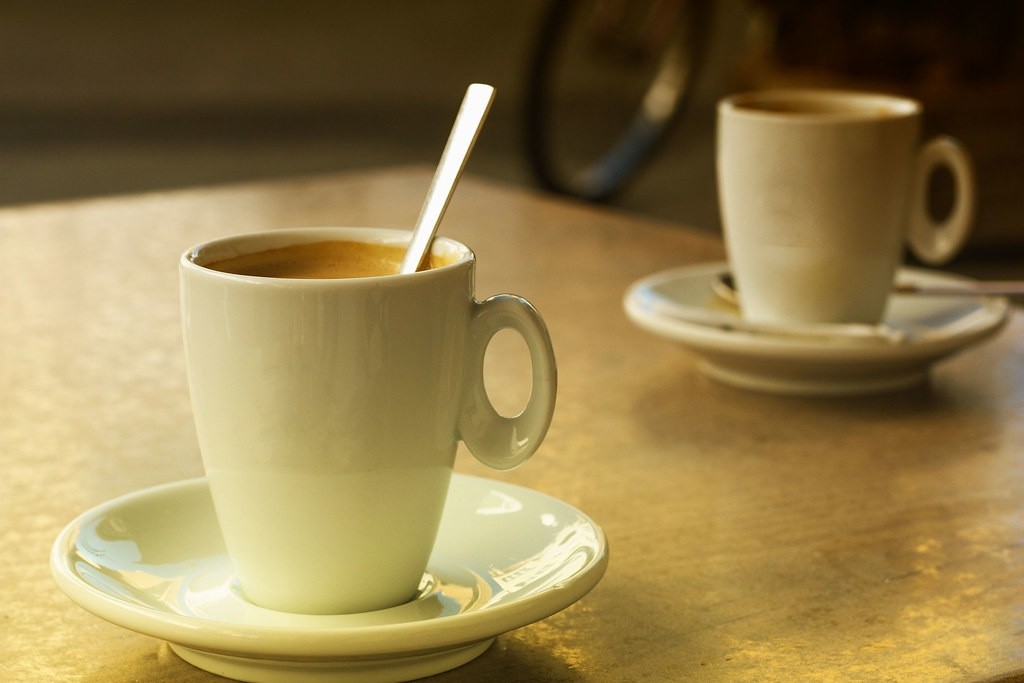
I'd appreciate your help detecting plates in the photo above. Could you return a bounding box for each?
[621,258,1010,399]
[51,477,610,683]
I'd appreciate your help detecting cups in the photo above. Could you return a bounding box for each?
[179,226,557,614]
[715,85,975,328]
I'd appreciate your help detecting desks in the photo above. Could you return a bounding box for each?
[0,166,1024,683]
